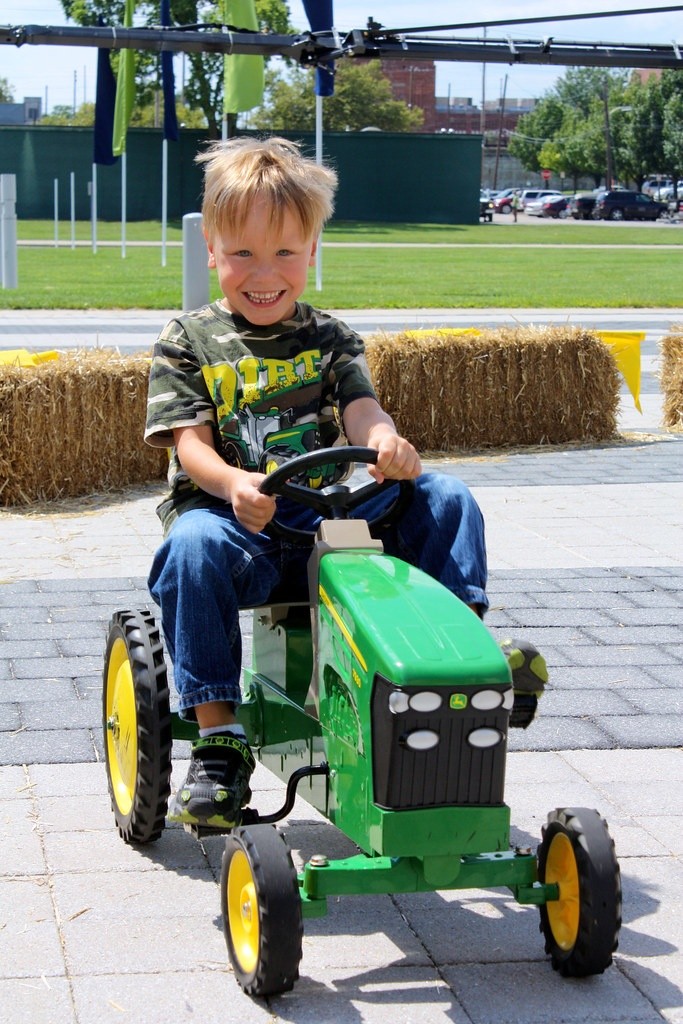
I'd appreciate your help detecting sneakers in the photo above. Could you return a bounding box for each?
[499,638,548,728]
[175,730,256,829]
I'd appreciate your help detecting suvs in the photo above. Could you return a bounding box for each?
[593,187,671,221]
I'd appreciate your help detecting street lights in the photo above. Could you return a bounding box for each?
[604,104,632,191]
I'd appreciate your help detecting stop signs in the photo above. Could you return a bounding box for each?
[542,170,552,179]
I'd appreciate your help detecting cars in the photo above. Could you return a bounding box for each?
[642,180,683,220]
[479,183,628,220]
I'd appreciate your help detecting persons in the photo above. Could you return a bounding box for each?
[148,139,548,834]
[512,191,520,222]
